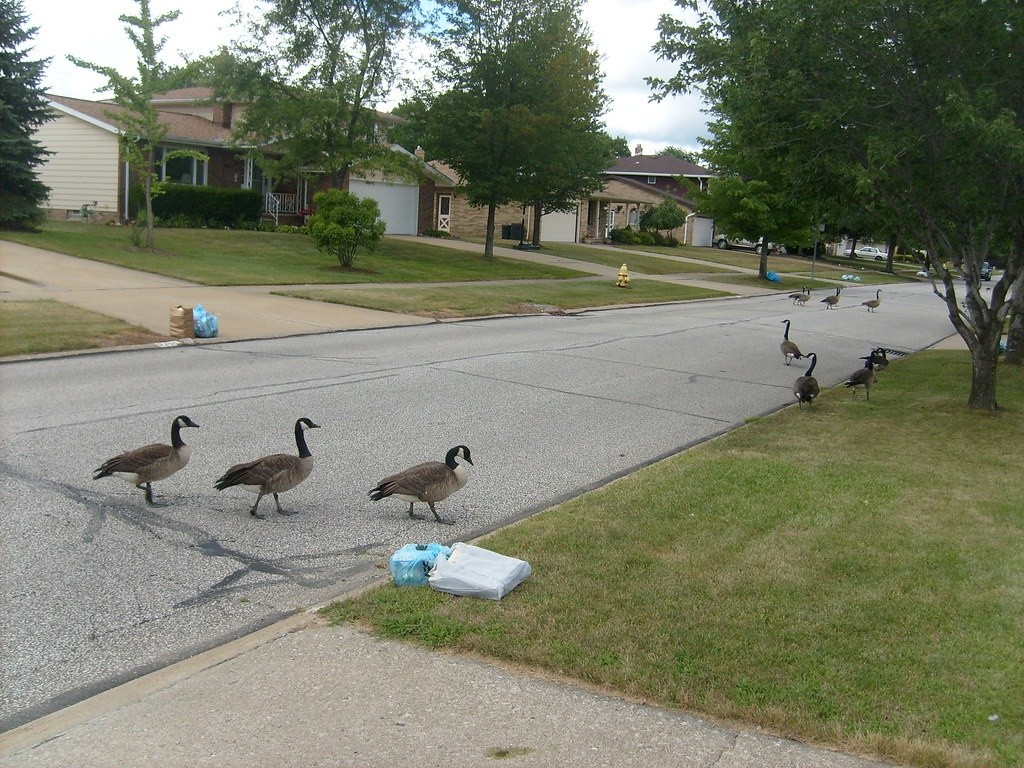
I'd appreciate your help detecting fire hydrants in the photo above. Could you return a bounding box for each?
[616,263,631,288]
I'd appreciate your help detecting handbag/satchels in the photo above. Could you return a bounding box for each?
[169,305,195,339]
[428,542,532,601]
[389,544,450,587]
[192,304,218,337]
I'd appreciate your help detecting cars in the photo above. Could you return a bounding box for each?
[844,247,888,262]
[713,233,826,257]
[980,262,992,281]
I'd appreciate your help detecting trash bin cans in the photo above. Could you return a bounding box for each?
[502,222,525,240]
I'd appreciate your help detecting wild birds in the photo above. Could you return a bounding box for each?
[780,319,821,409]
[367,445,474,525]
[820,286,842,310]
[844,348,889,401]
[789,286,812,307]
[860,288,883,313]
[91,415,200,509]
[209,418,322,519]
